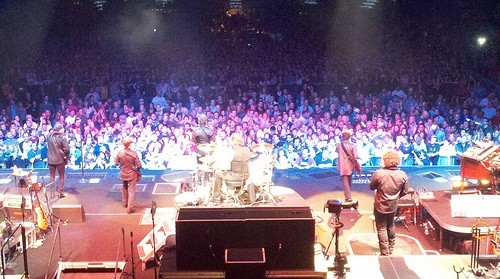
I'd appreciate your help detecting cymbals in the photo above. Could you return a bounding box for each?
[197,144,215,152]
[250,144,273,151]
[198,157,214,163]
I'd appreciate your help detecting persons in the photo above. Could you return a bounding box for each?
[0,0,500,172]
[209,133,249,203]
[369,149,409,256]
[335,130,361,202]
[27,172,54,239]
[114,136,142,214]
[47,124,70,199]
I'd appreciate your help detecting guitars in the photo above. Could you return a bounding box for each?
[32,185,49,232]
[58,148,68,165]
[105,151,141,182]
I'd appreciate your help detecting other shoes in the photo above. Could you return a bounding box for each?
[123,203,128,208]
[128,209,136,214]
[51,193,56,199]
[346,198,352,202]
[389,251,393,254]
[58,192,68,199]
[381,252,389,256]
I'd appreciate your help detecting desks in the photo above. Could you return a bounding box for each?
[394,199,416,230]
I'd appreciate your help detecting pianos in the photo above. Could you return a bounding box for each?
[460,142,500,193]
